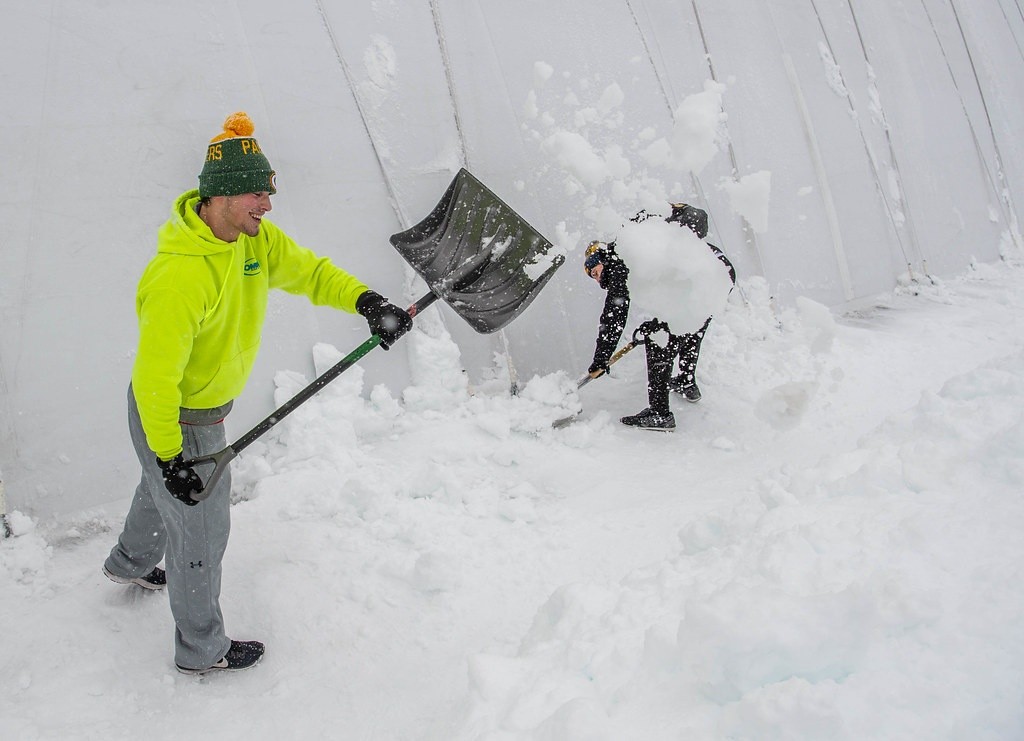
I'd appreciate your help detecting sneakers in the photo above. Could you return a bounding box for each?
[670,375,701,403]
[175,640,265,675]
[101,564,167,591]
[620,408,676,431]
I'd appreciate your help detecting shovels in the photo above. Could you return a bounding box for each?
[159,166,569,503]
[509,327,646,431]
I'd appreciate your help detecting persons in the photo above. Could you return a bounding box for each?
[101,111,414,676]
[584,201,736,431]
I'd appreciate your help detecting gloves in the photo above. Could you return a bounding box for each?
[355,290,414,350]
[156,451,205,506]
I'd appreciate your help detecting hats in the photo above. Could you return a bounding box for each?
[584,241,607,278]
[200,113,276,196]
